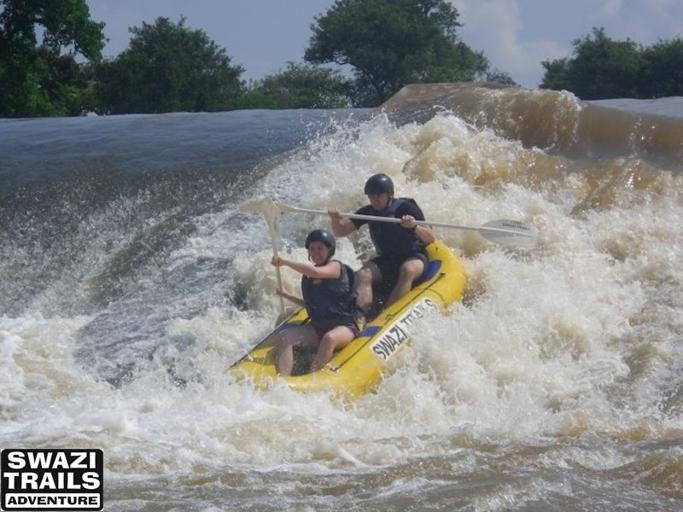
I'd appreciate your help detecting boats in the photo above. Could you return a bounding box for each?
[224,239,467,410]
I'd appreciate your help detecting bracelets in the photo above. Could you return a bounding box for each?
[411,225,417,232]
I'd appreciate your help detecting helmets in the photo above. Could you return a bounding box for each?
[304,229,336,249]
[365,174,394,193]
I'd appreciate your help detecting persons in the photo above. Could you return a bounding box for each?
[328,173,436,314]
[272,229,366,377]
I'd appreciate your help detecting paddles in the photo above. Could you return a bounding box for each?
[239,199,538,247]
[261,197,295,329]
